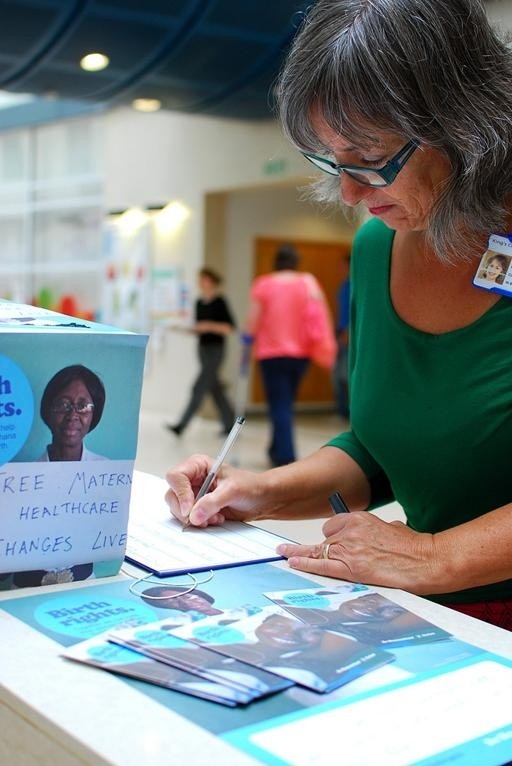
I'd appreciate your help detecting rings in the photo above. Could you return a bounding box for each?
[324,542,331,558]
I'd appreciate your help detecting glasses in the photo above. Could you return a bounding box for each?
[49,400,99,415]
[287,122,418,188]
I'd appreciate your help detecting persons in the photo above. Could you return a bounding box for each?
[481,252,508,285]
[165,265,239,437]
[0,362,115,589]
[283,592,409,633]
[241,238,339,466]
[330,253,350,419]
[140,585,231,619]
[211,613,324,689]
[165,0,512,595]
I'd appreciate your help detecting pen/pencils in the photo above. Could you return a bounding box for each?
[181,416,246,533]
[328,492,350,514]
